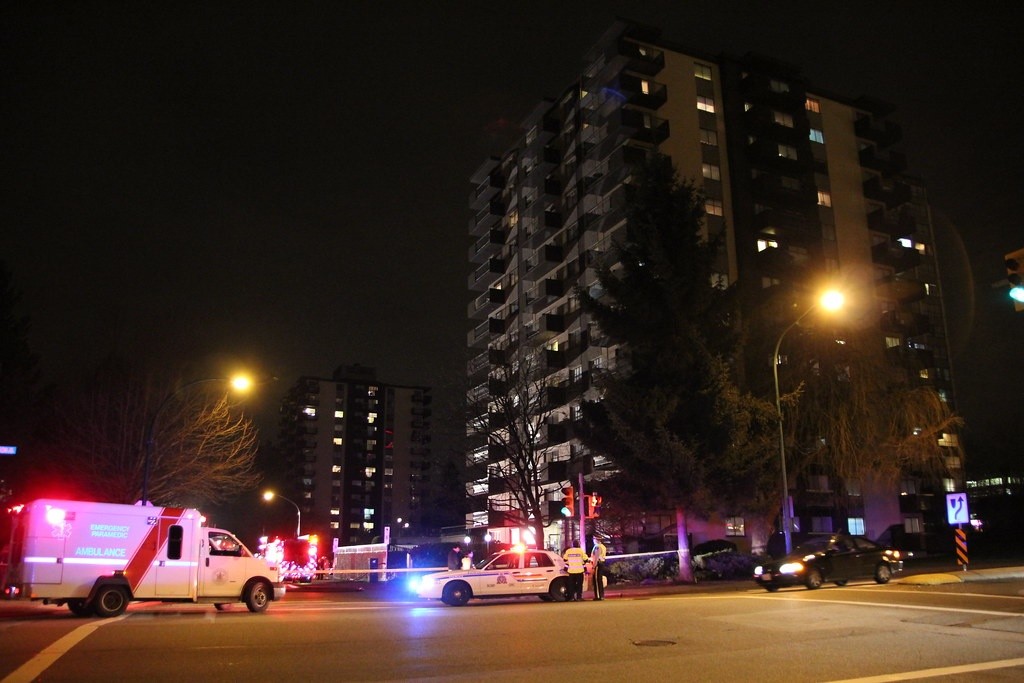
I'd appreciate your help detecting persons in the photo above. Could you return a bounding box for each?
[316,559,324,580]
[590,534,606,601]
[448,544,476,572]
[563,539,588,602]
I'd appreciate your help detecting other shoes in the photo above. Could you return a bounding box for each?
[570,598,575,601]
[593,597,604,601]
[577,598,584,601]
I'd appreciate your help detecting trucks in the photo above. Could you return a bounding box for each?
[4,498,286,618]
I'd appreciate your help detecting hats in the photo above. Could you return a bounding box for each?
[592,533,603,539]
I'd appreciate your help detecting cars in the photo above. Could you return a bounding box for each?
[417,545,608,607]
[752,533,904,593]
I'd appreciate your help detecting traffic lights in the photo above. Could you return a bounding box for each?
[1004,248,1024,312]
[562,486,574,517]
[589,496,602,517]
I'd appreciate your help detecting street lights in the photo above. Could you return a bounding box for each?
[265,492,301,537]
[143,376,248,506]
[773,292,842,554]
